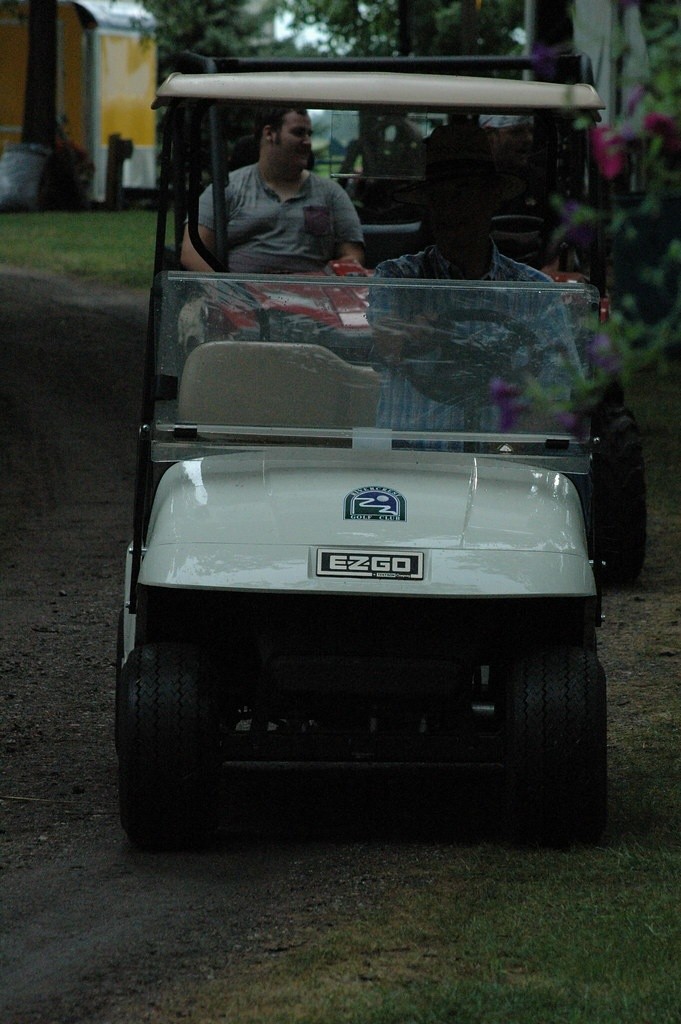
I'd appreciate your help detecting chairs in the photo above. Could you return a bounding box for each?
[175,337,378,433]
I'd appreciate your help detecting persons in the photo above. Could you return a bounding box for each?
[177,108,367,384]
[231,107,425,186]
[371,126,580,453]
[480,112,615,296]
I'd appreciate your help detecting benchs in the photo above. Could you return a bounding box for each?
[356,215,548,265]
